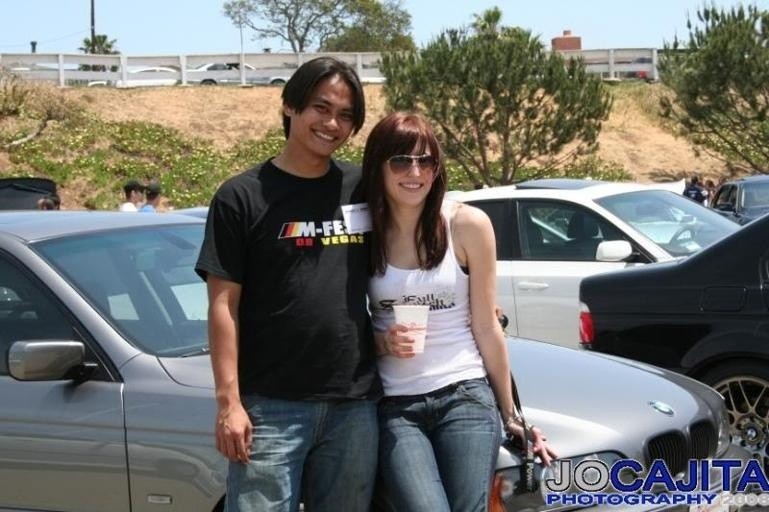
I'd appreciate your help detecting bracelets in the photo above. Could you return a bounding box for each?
[502,417,520,429]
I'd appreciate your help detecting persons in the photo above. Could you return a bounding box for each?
[120,181,145,212]
[363,113,556,512]
[38,198,54,209]
[195,55,502,512]
[683,176,715,207]
[138,183,161,213]
[47,196,60,210]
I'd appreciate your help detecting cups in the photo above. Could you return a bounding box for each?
[392,304,430,354]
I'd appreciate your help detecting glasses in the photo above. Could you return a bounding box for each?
[385,153,437,175]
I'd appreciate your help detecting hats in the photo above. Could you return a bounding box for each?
[145,183,161,193]
[124,180,149,191]
